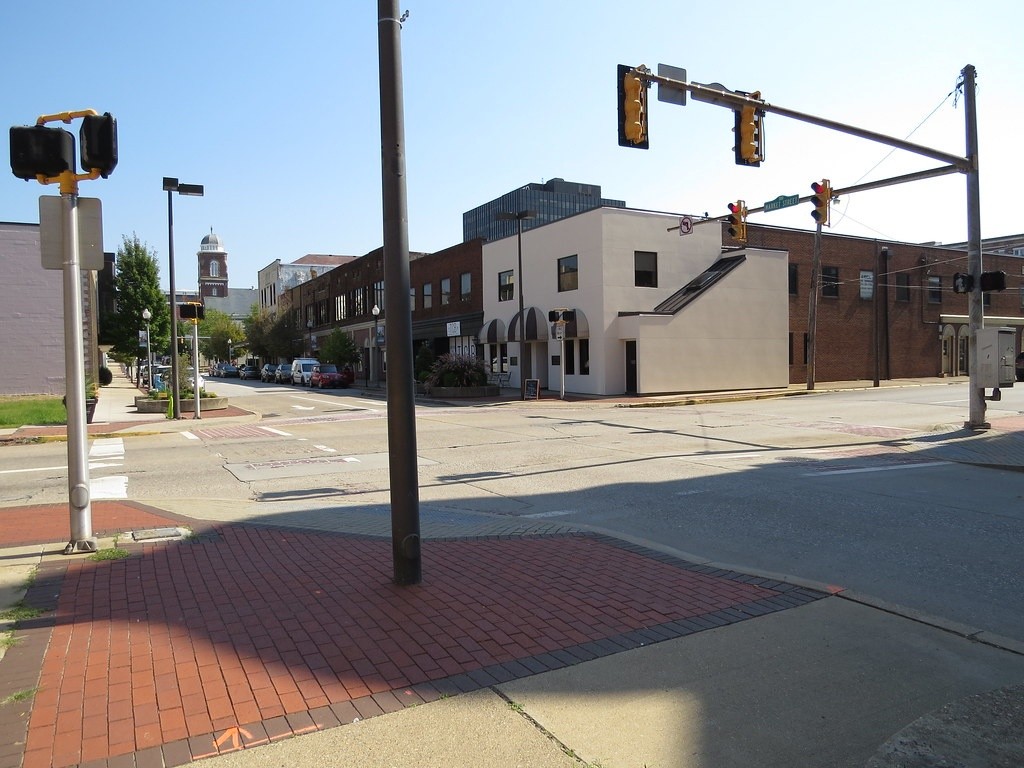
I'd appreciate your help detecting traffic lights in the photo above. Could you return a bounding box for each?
[199,305,206,320]
[180,304,197,318]
[810,179,828,224]
[561,310,576,322]
[953,274,975,295]
[724,200,744,239]
[549,312,559,322]
[80,112,119,178]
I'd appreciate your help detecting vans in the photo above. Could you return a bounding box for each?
[291,357,321,386]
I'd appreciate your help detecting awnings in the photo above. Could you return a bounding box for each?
[508,306,548,341]
[550,309,590,339]
[476,319,506,344]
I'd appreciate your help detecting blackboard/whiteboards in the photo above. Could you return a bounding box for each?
[524,379,539,399]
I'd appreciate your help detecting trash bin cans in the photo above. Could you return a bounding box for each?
[155,374,169,393]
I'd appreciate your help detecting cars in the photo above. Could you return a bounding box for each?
[261,364,278,382]
[239,366,259,380]
[309,364,348,389]
[276,365,291,381]
[208,364,237,378]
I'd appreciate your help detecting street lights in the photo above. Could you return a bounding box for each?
[372,305,380,392]
[227,338,232,365]
[164,178,205,421]
[496,210,535,400]
[142,308,154,394]
